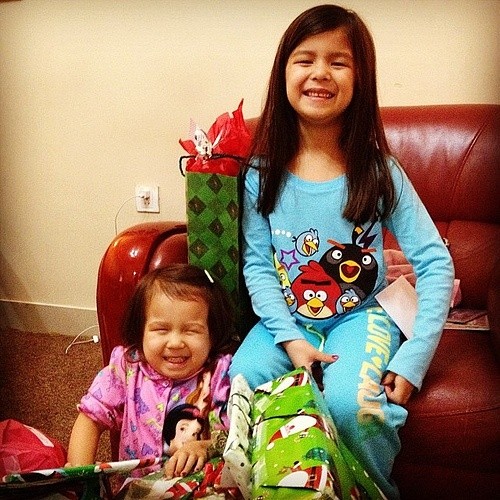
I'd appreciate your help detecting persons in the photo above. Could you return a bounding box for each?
[227,5,456,500]
[63,264,232,500]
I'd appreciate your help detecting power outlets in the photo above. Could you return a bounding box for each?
[135,186,159,213]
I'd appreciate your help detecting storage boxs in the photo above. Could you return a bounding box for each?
[186,170,256,334]
[251,367,390,500]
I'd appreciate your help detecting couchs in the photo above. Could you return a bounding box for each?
[97,104,500,500]
